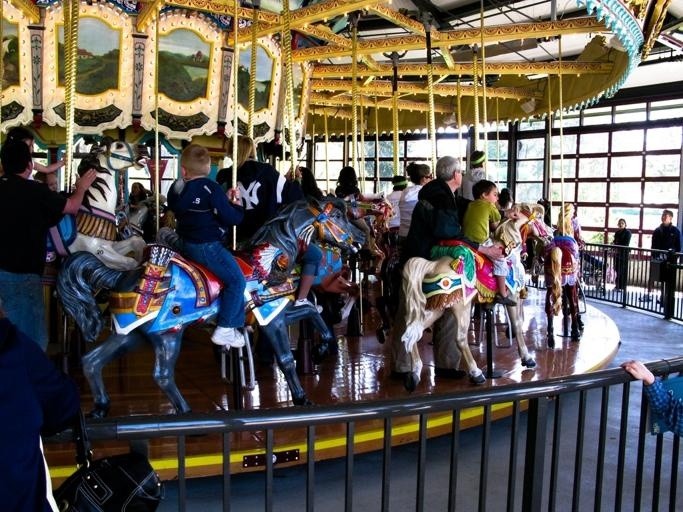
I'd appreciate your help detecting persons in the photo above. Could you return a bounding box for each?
[297,167,322,201]
[0,141,97,353]
[34,171,58,192]
[389,156,520,382]
[463,179,517,306]
[129,183,147,205]
[167,144,246,348]
[335,166,370,238]
[1,126,67,174]
[397,162,432,264]
[620,359,682,439]
[459,150,485,200]
[0,296,79,512]
[216,135,323,315]
[389,175,408,246]
[610,218,631,292]
[650,210,681,304]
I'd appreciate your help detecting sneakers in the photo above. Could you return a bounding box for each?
[493,292,517,308]
[431,367,465,380]
[294,298,324,317]
[210,326,246,349]
[388,372,408,384]
[639,294,652,302]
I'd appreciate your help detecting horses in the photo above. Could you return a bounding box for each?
[55,195,367,420]
[400,203,554,395]
[46,137,147,272]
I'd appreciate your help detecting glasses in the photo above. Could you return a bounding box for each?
[424,173,432,179]
